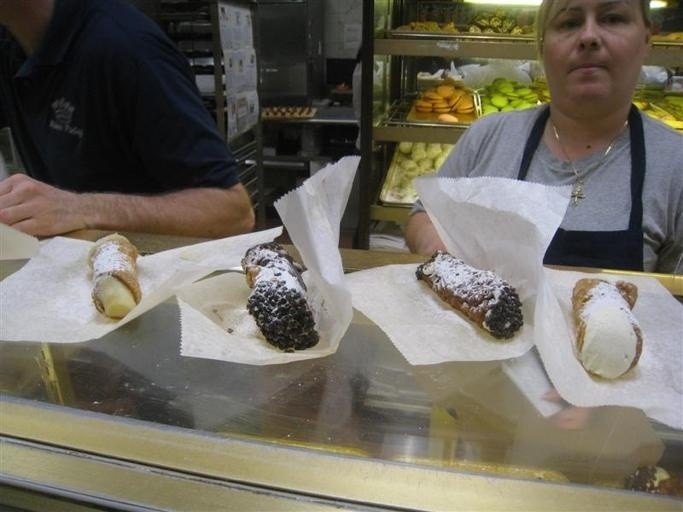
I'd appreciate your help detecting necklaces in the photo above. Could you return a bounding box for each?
[550,119,631,206]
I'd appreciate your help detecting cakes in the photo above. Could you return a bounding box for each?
[416,250,522,339]
[416,101,432,113]
[400,144,453,172]
[482,75,537,113]
[470,8,525,40]
[455,89,466,95]
[436,85,454,97]
[241,241,319,352]
[87,233,141,318]
[571,277,642,377]
[425,88,436,98]
[428,95,442,105]
[440,113,457,122]
[450,95,463,110]
[434,103,450,113]
[457,103,474,113]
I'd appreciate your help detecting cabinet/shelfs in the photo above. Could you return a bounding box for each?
[148,0,267,232]
[351,0,683,251]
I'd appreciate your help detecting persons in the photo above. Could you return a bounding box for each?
[1,1,255,237]
[407,0,682,276]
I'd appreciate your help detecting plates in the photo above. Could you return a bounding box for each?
[385,1,682,46]
[475,88,540,118]
[405,88,480,128]
[377,141,456,209]
